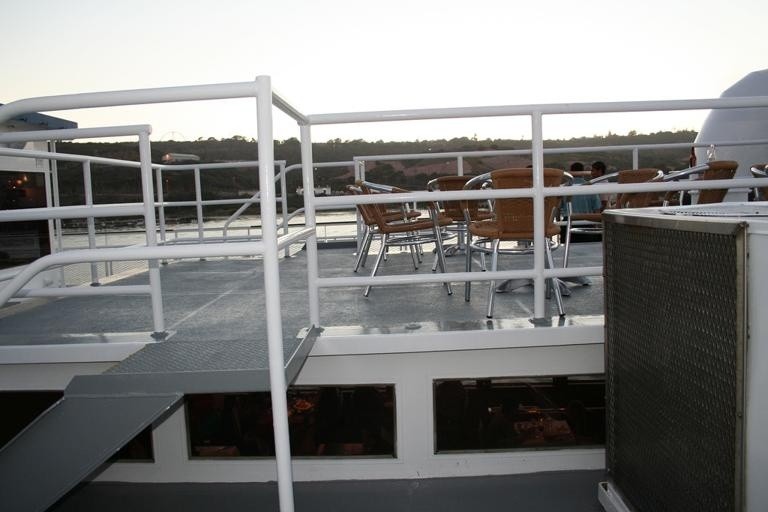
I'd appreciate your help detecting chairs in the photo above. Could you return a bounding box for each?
[428,177,493,271]
[662,159,738,207]
[461,171,571,318]
[355,179,454,299]
[747,163,768,201]
[553,167,662,270]
[347,184,423,272]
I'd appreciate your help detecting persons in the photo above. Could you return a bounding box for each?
[556,162,602,242]
[567,399,592,444]
[517,165,532,247]
[347,387,386,442]
[591,162,612,212]
[487,398,520,448]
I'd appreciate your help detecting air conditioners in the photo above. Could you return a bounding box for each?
[596,199,766,509]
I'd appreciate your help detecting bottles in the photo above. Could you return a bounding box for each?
[690,146,696,167]
